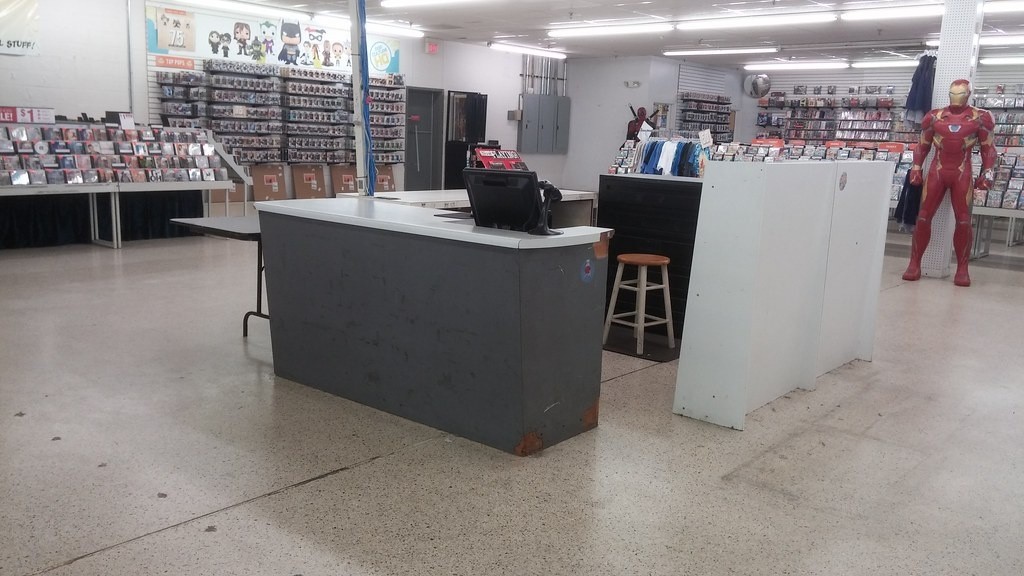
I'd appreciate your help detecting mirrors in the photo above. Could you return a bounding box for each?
[744,73,771,98]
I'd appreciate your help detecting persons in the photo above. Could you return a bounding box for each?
[900,79,997,287]
[752,80,759,95]
[620,107,656,153]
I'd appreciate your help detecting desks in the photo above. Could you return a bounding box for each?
[606,97,1024,261]
[170,218,270,337]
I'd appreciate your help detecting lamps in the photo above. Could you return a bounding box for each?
[660,46,781,57]
[488,42,568,60]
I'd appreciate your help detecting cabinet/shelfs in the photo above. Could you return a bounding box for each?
[156,62,407,165]
[0,122,253,249]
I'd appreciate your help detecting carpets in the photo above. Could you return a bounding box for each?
[604,328,680,362]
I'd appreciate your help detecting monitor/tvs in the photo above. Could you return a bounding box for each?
[463,167,544,233]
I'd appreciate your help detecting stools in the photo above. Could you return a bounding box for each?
[602,253,675,354]
